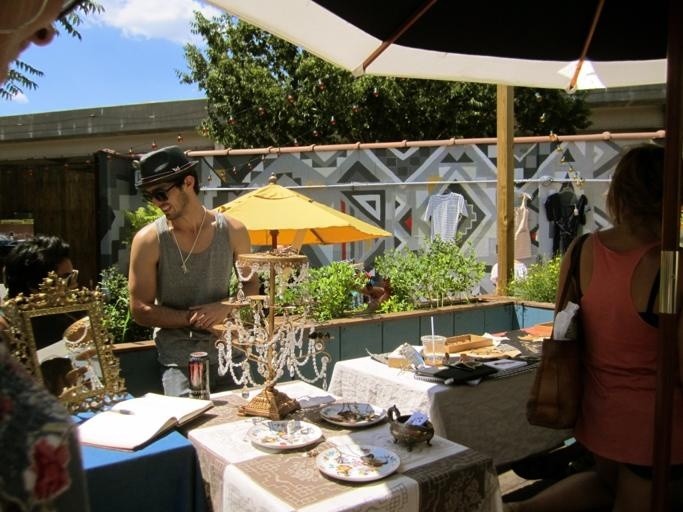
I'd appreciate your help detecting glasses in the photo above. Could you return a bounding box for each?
[137,182,176,206]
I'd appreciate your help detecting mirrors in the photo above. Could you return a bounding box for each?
[0,269,127,414]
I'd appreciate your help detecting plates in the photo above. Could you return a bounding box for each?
[248,418,323,451]
[319,402,387,428]
[314,444,401,485]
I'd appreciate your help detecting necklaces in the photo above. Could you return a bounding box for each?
[169,210,216,278]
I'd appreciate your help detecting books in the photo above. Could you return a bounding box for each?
[70,388,213,450]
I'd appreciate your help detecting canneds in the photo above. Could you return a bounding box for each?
[189,351,211,400]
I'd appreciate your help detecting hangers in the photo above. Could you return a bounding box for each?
[432,181,453,195]
[561,178,575,193]
[513,178,533,200]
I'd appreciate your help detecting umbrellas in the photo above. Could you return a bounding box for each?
[193,171,377,381]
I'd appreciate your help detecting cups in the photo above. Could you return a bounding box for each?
[420,333,449,367]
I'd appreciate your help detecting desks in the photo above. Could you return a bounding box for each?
[328,321,576,465]
[181,377,503,512]
[64,392,196,512]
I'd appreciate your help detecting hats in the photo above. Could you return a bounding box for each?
[135,145,201,188]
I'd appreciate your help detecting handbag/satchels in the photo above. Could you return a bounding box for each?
[524,232,590,432]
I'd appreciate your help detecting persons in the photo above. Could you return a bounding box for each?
[124,146,252,398]
[0,231,80,346]
[493,142,664,510]
